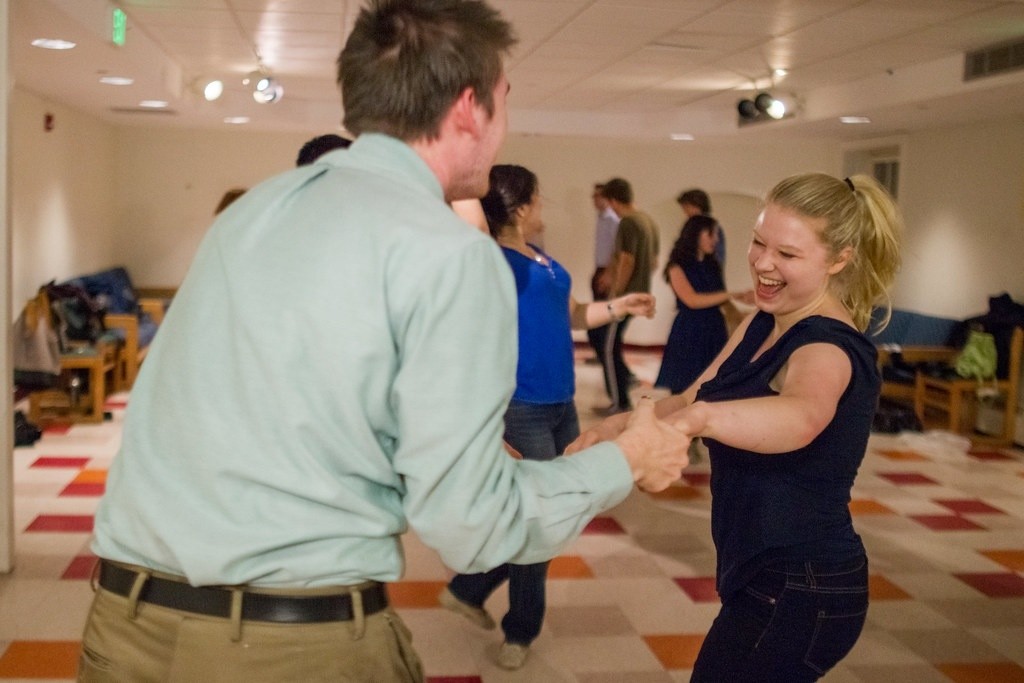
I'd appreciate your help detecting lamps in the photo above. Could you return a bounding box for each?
[764,94,807,119]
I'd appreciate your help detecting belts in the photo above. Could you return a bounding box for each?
[99,560,389,625]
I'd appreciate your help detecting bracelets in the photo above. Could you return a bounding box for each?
[608,302,624,323]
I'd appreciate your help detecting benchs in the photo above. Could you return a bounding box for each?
[866,304,969,409]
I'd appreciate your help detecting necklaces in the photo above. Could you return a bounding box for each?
[535,254,544,262]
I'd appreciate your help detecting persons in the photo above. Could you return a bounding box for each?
[73,0,691,683]
[653,213,754,463]
[436,163,657,672]
[678,188,728,270]
[564,173,905,682]
[212,134,354,221]
[592,177,662,418]
[585,184,622,363]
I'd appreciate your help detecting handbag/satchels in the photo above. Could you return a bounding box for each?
[956,331,998,383]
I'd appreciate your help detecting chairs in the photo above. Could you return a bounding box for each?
[24,283,180,428]
[914,324,1023,448]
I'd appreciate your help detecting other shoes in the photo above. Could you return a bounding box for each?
[437,586,497,631]
[497,641,529,669]
[591,403,635,416]
